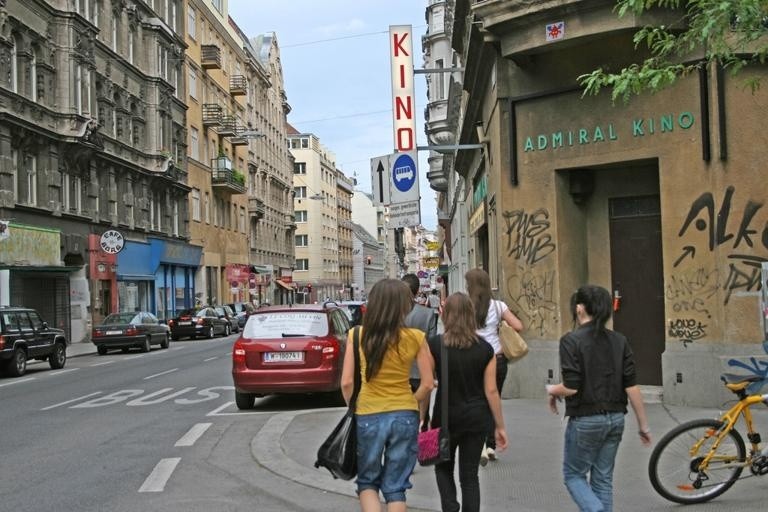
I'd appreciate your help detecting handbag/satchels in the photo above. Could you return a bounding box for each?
[316,411,357,482]
[416,427,454,466]
[496,321,530,362]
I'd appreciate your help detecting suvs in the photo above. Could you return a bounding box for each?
[0,304,70,378]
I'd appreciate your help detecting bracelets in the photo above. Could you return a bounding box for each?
[639,427,651,434]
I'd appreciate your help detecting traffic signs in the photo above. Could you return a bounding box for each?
[385,199,424,229]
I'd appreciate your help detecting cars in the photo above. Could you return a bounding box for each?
[327,301,368,328]
[163,299,264,339]
[234,302,353,409]
[88,309,174,356]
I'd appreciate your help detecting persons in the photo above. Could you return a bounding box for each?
[428,291,509,512]
[341,279,435,512]
[425,289,442,330]
[464,269,523,467]
[417,293,426,304]
[401,274,437,432]
[546,284,651,512]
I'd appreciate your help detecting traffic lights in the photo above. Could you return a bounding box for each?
[308,283,312,293]
[367,256,371,265]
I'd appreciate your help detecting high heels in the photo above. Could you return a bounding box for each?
[480,447,498,467]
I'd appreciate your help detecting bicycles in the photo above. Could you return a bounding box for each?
[646,368,768,507]
